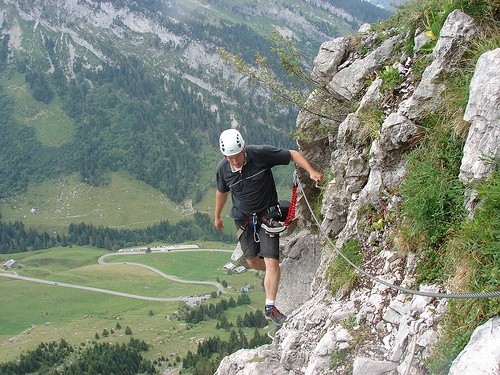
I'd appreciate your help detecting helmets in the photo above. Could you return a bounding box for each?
[219,128,245,157]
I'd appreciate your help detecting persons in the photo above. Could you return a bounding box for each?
[214,129,322,325]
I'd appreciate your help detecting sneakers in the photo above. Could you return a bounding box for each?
[265,306,287,325]
